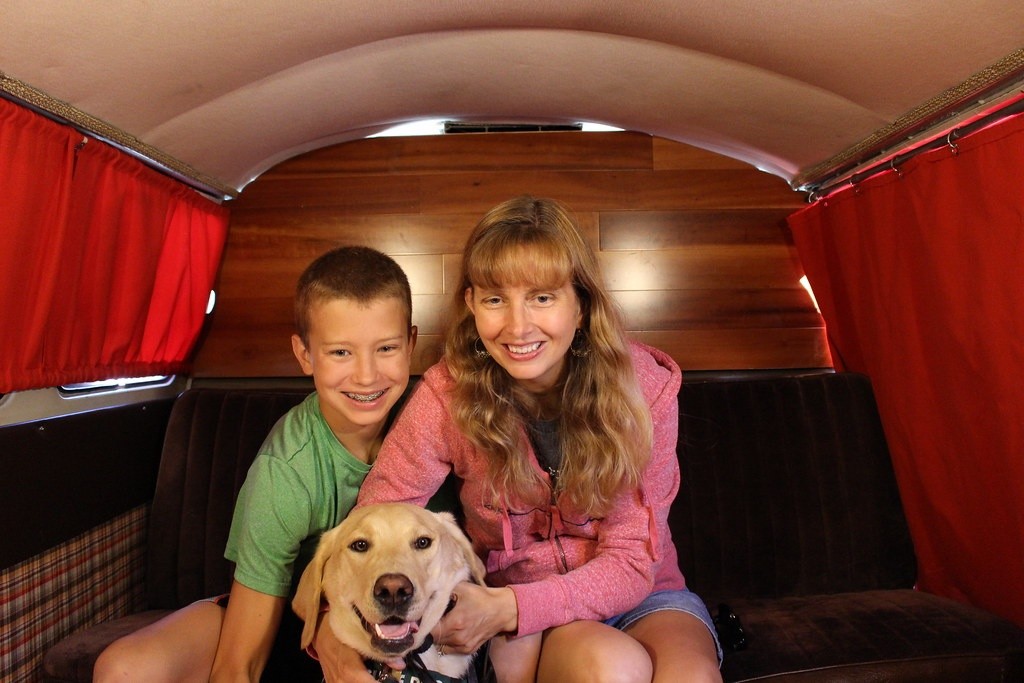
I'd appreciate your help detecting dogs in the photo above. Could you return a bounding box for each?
[290,502,489,683]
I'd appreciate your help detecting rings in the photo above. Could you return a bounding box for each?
[437,643,447,659]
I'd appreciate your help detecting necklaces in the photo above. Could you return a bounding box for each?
[525,422,562,480]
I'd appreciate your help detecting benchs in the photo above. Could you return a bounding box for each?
[42,369,1024,683]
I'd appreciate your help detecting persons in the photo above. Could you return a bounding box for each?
[92,245,472,683]
[348,193,724,683]
